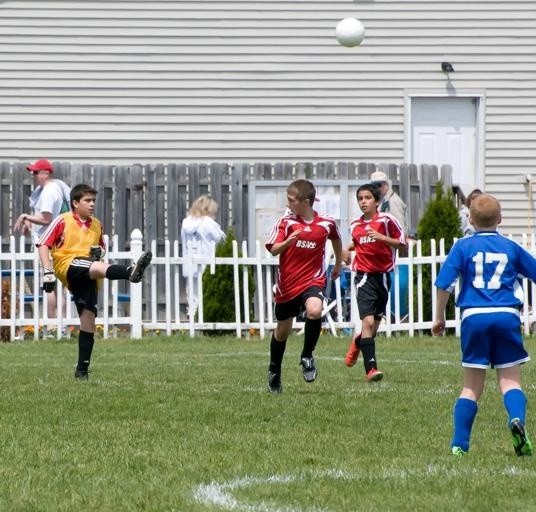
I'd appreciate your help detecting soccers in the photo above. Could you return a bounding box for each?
[336,18,364,47]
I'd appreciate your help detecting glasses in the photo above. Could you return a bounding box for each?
[32,170,45,175]
[375,181,385,187]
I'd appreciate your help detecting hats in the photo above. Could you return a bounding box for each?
[25,159,52,173]
[369,171,388,183]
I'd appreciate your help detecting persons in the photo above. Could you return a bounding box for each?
[371,172,408,323]
[458,189,484,236]
[342,184,408,383]
[13,159,70,339]
[265,179,342,396]
[431,194,536,458]
[180,195,227,319]
[35,184,152,380]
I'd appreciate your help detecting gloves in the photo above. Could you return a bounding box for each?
[41,270,55,293]
[88,246,105,260]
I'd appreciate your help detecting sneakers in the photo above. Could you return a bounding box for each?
[267,365,282,396]
[300,351,317,383]
[60,326,66,337]
[38,328,55,339]
[125,250,151,283]
[450,445,469,458]
[345,332,361,367]
[73,367,88,382]
[366,367,383,382]
[508,417,533,458]
